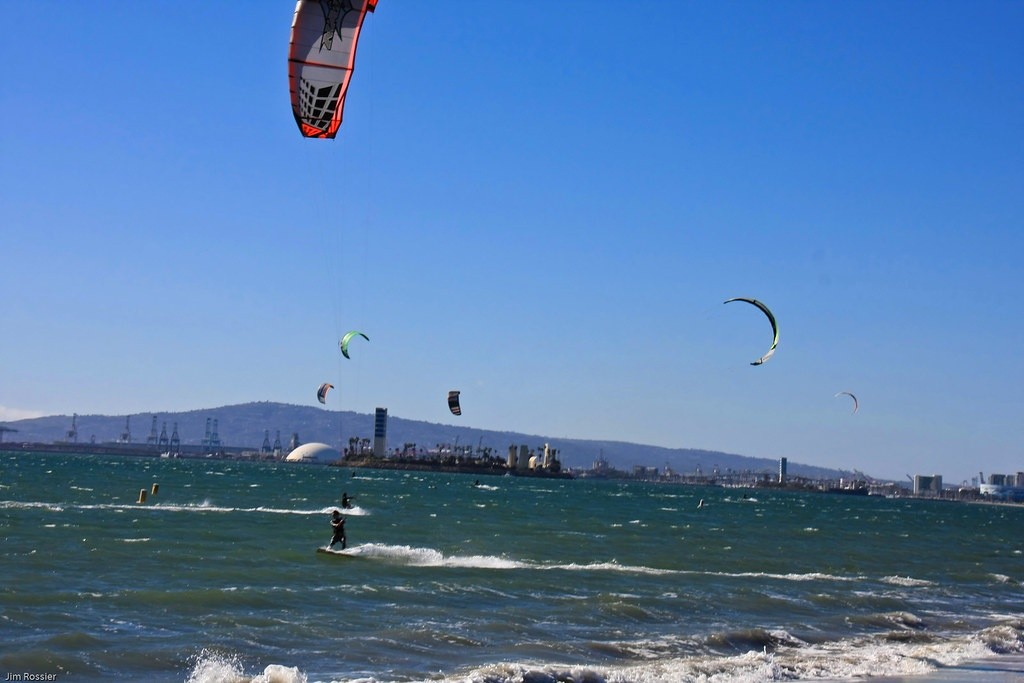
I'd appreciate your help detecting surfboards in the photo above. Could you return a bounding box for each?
[317,547,355,557]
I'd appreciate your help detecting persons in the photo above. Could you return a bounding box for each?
[324,510,347,550]
[342,493,355,508]
